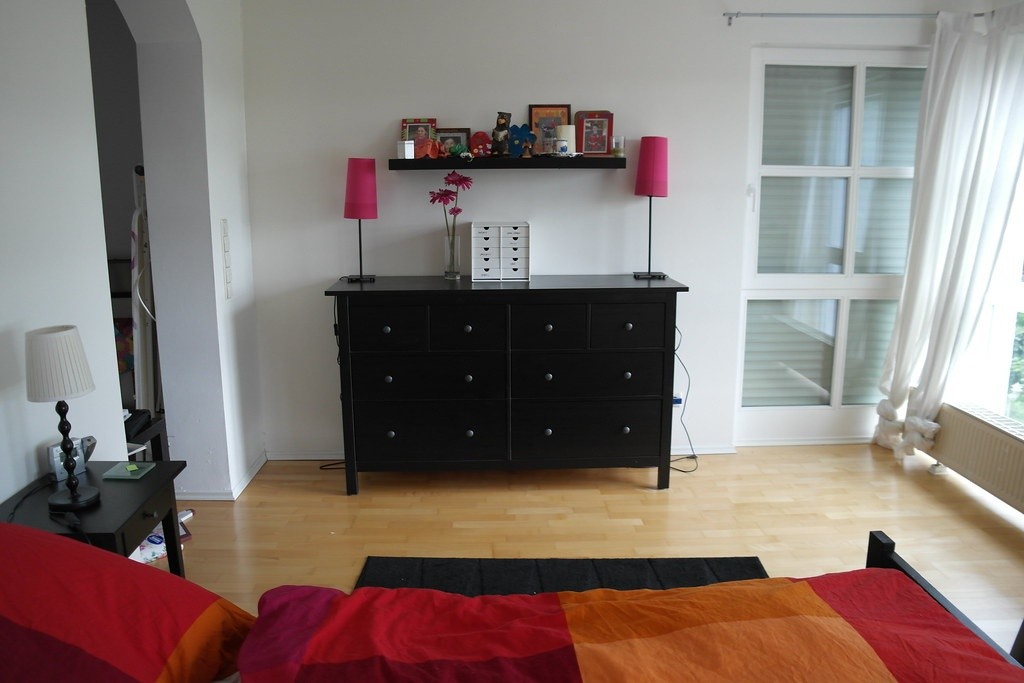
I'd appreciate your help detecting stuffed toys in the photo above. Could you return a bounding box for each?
[491,111,512,154]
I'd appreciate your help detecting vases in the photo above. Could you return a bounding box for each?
[443,235,461,280]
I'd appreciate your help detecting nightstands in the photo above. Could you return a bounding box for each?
[0,461,188,579]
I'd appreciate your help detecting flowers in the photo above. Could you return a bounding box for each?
[428,169,473,273]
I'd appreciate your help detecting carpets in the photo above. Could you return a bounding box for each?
[349,554,769,597]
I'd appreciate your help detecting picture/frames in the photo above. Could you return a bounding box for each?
[435,128,471,150]
[576,111,613,156]
[528,104,571,157]
[401,118,437,144]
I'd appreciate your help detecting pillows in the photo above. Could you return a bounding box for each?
[0,522,257,683]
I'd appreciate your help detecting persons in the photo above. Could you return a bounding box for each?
[586,123,603,151]
[541,119,557,137]
[415,126,428,144]
[438,137,456,156]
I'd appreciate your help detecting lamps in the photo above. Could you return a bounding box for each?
[344,158,378,283]
[633,136,669,280]
[25,325,101,511]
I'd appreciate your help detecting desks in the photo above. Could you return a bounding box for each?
[125,414,171,463]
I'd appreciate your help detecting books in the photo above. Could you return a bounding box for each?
[127,442,147,456]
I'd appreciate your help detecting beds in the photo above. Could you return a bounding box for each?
[0,520,1024,683]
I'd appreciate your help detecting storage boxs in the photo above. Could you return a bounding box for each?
[470,222,531,284]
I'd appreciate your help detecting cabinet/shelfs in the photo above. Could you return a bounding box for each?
[323,273,689,490]
